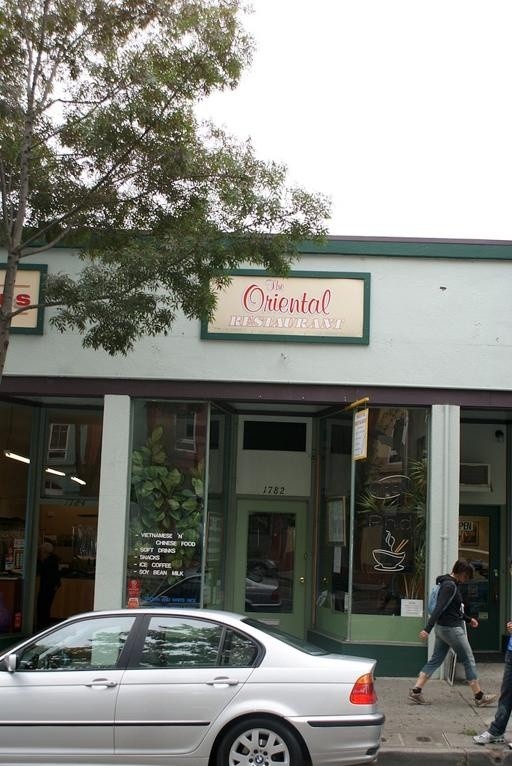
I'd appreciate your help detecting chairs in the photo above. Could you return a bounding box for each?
[138,639,167,668]
[228,644,257,668]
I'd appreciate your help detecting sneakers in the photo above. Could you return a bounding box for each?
[472,730,505,744]
[408,688,432,705]
[472,693,499,707]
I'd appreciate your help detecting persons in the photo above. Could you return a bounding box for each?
[472,621,512,751]
[408,558,498,708]
[35,542,62,635]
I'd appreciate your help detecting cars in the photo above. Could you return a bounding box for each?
[134,570,284,612]
[0,608,387,766]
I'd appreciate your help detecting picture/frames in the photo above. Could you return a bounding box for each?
[323,495,346,548]
[458,518,480,549]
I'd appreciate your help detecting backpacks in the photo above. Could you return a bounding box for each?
[428,580,457,624]
